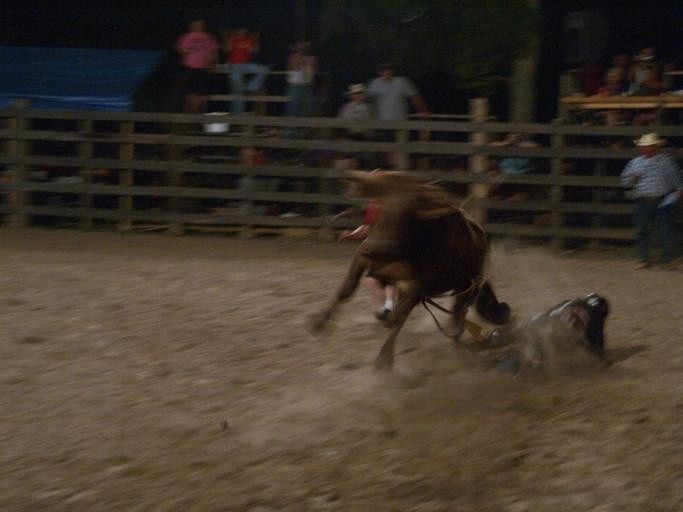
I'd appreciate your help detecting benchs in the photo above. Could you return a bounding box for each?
[559,94,683,110]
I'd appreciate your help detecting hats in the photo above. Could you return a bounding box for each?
[348,83,366,94]
[633,134,665,146]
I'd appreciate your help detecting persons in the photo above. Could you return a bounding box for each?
[128,27,680,250]
[464,292,613,376]
[337,168,413,329]
[619,133,681,271]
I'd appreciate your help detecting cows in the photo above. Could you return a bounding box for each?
[304,169,490,375]
